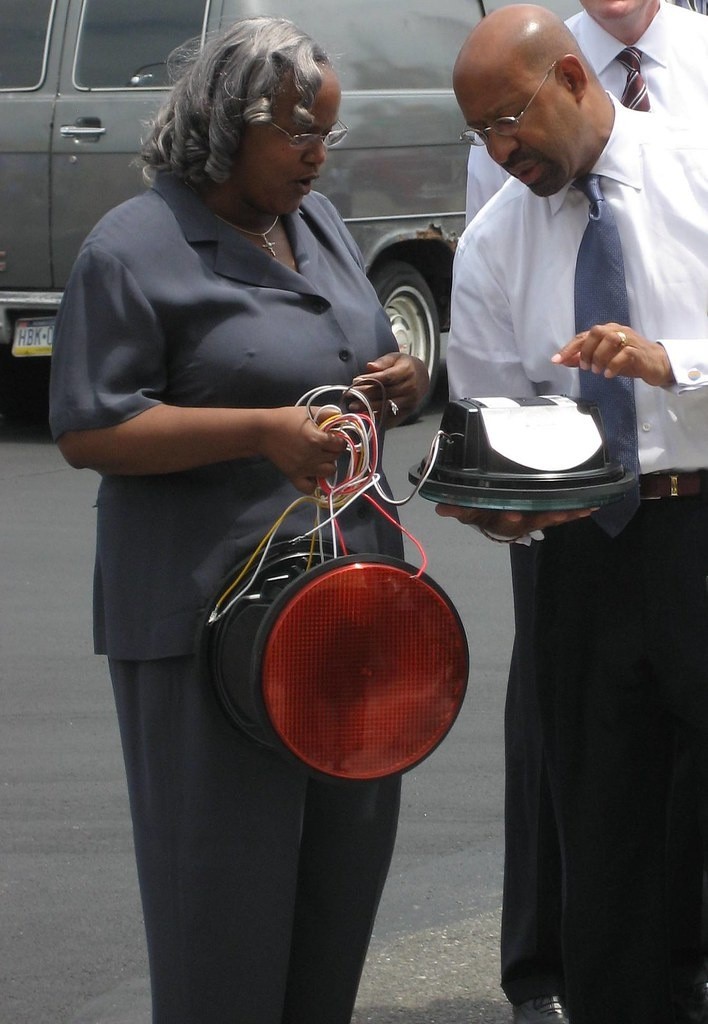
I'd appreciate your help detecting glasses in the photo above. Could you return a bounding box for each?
[271,120,349,149]
[458,60,557,146]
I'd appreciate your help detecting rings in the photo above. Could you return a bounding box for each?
[388,399,399,415]
[617,332,627,348]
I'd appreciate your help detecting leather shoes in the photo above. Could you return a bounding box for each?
[512,995,569,1024]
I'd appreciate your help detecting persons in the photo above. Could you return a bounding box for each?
[465,1,708,1024]
[435,3,708,1024]
[49,18,430,1024]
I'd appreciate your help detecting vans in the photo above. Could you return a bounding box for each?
[0,1,588,423]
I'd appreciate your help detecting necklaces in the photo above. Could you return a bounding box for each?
[215,215,279,257]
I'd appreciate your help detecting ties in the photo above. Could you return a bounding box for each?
[572,173,640,538]
[616,46,650,112]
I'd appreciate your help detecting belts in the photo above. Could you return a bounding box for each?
[639,472,707,500]
